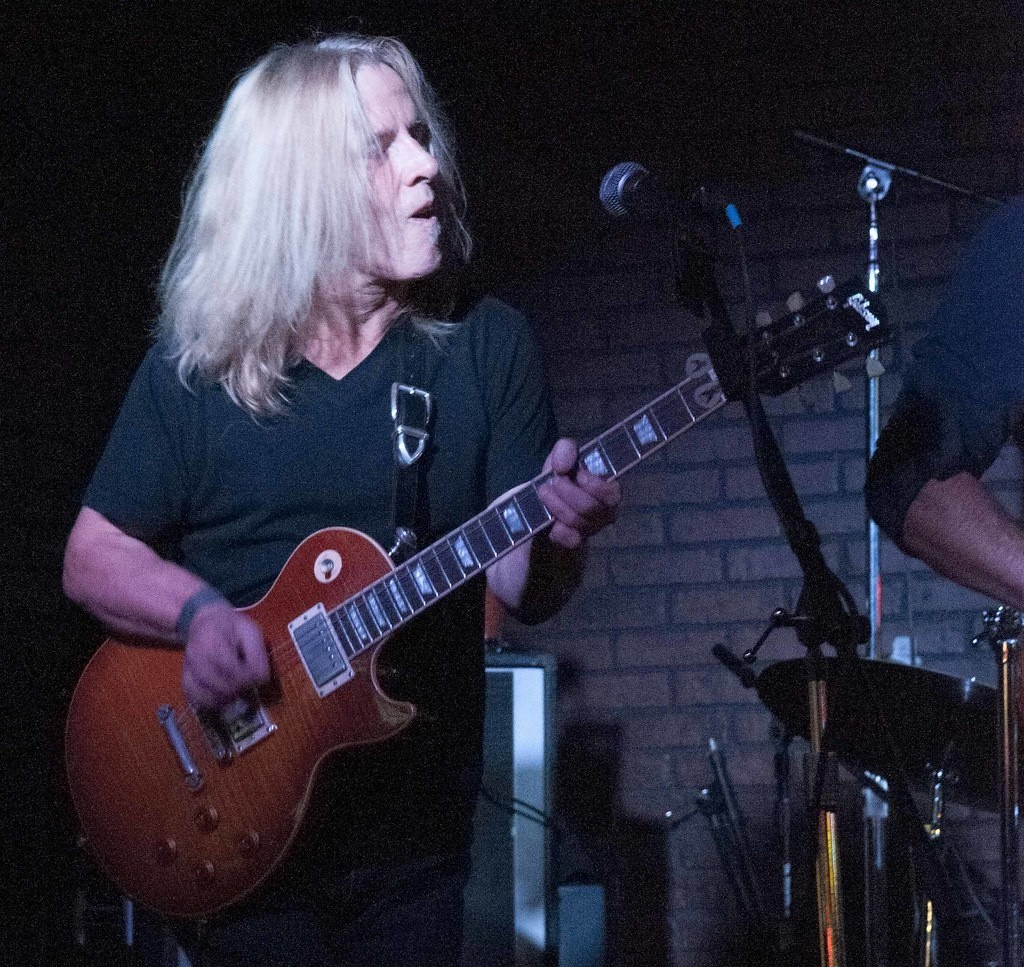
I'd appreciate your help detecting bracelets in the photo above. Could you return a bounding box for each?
[176,588,222,648]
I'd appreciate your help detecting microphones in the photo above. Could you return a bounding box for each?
[792,126,976,206]
[599,158,679,219]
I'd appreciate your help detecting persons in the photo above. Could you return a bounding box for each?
[864,197,1024,614]
[61,37,623,967]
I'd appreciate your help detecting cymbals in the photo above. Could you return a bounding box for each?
[756,655,1024,813]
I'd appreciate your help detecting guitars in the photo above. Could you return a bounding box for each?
[64,275,890,921]
